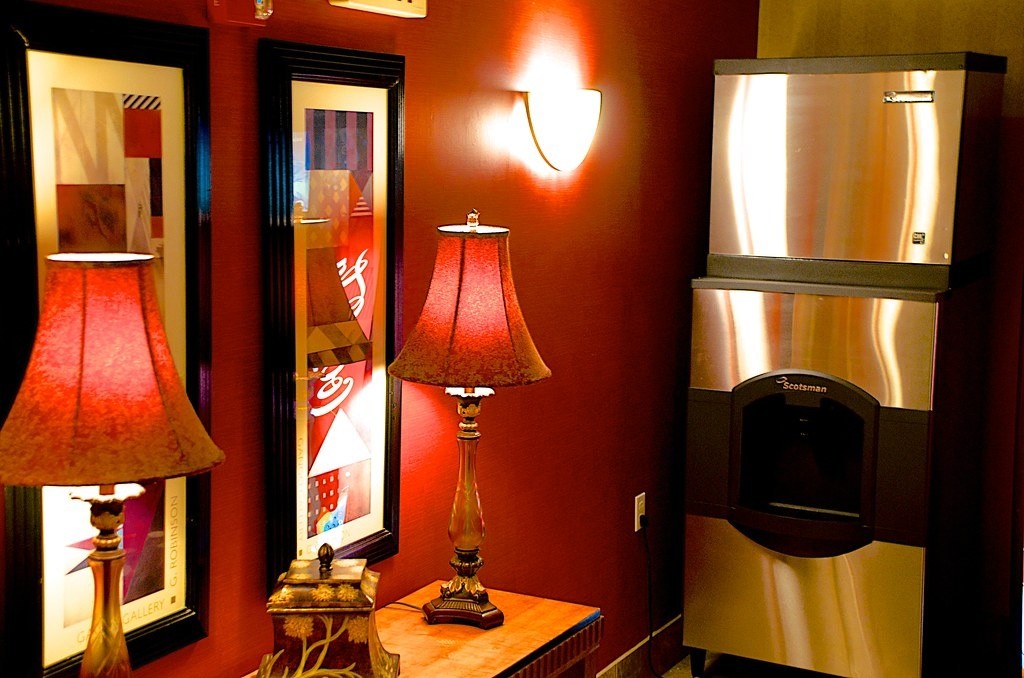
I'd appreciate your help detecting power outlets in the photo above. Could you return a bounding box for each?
[635,492,646,531]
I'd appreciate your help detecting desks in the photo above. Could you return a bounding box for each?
[239,579,614,678]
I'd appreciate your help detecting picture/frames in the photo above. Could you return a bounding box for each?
[0,1,212,678]
[257,36,398,602]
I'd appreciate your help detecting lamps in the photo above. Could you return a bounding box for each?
[0,253,229,678]
[250,540,403,678]
[511,87,602,175]
[388,209,550,630]
